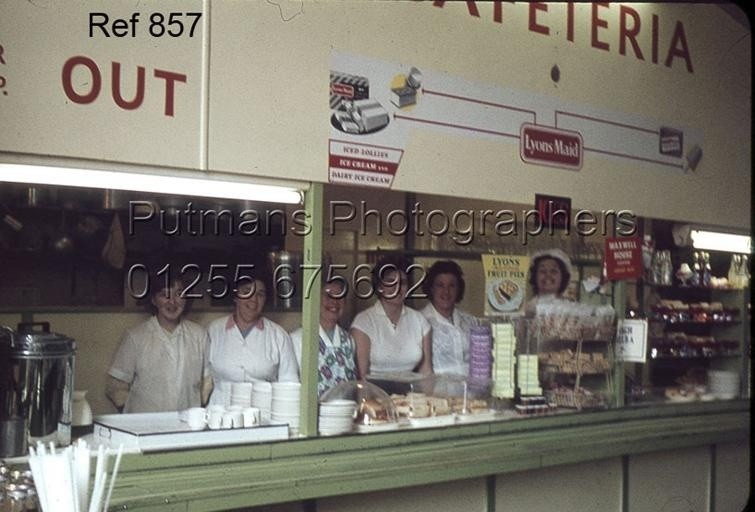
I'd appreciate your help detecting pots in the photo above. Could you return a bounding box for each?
[0,320,78,459]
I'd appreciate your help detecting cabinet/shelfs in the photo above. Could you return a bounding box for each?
[625,276,742,404]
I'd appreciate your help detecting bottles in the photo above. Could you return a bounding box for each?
[0,460,39,512]
[652,249,750,291]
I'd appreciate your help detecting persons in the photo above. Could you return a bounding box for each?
[288,279,358,401]
[414,259,481,376]
[102,274,213,414]
[350,256,435,397]
[204,269,299,381]
[520,247,577,314]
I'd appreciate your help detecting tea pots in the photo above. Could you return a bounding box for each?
[73,388,94,436]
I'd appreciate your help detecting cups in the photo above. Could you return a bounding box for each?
[179,404,220,431]
[656,304,743,358]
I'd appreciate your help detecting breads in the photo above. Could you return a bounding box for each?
[358,391,489,425]
[494,279,518,305]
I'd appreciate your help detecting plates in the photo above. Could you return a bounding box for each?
[662,388,698,402]
[316,399,358,439]
[487,279,524,313]
[220,381,299,437]
[706,370,739,400]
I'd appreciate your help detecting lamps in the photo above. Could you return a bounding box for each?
[669,223,753,256]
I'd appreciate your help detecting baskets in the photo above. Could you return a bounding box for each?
[526,321,617,342]
[541,388,608,409]
[540,357,605,377]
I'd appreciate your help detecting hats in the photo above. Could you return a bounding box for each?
[532,248,573,277]
